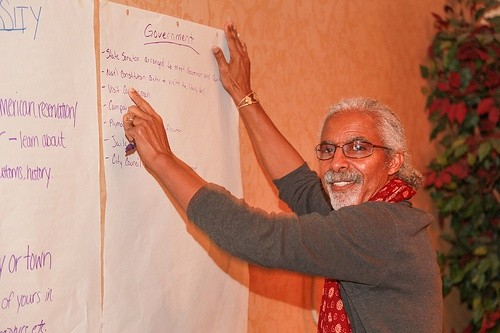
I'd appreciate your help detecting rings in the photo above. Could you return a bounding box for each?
[127,115,136,126]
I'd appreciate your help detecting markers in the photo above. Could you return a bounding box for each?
[125,140,136,152]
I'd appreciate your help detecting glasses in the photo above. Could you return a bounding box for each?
[315,142,392,161]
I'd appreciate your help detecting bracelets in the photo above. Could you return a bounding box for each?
[236,91,260,110]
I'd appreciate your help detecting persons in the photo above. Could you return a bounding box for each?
[123,24,444,333]
